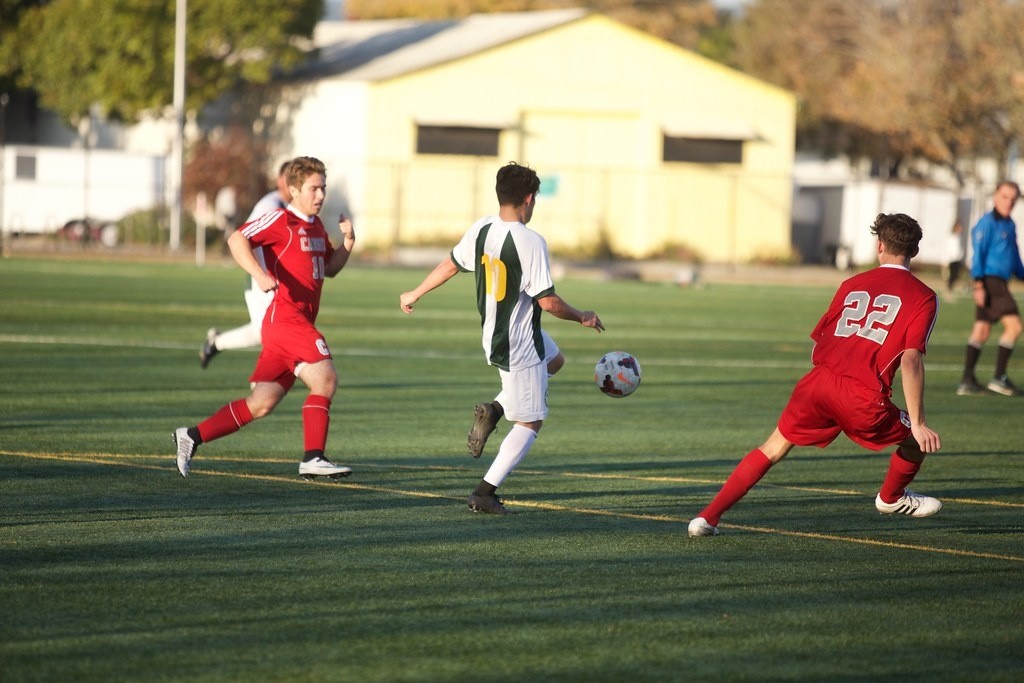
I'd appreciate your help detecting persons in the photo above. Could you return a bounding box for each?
[171,156,356,478]
[947,221,964,290]
[198,160,291,370]
[688,214,944,540]
[400,159,606,516]
[954,180,1024,397]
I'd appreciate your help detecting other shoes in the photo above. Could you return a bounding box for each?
[957,384,990,397]
[988,379,1024,397]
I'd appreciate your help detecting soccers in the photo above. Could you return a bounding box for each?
[593,351,642,399]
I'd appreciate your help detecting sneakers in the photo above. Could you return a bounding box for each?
[200,328,220,369]
[467,403,496,458]
[171,426,197,478]
[298,457,353,481]
[875,488,943,518]
[468,493,516,514]
[688,517,720,538]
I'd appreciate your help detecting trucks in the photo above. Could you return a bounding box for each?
[0,143,163,248]
